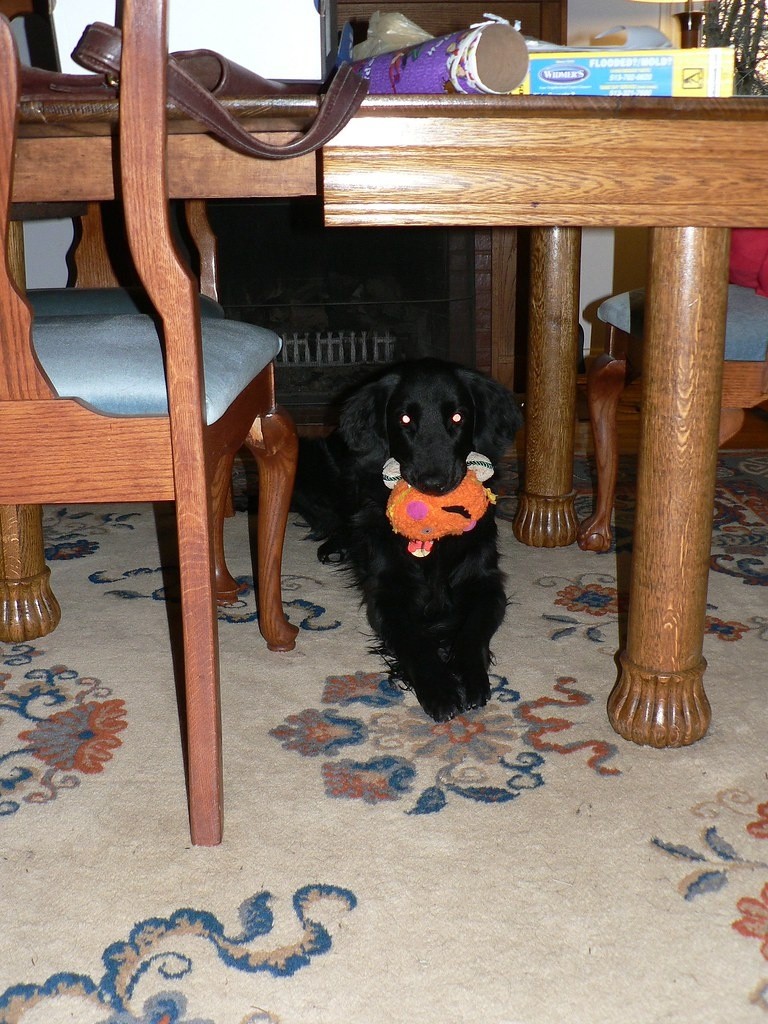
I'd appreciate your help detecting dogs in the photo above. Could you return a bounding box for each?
[285,356,522,724]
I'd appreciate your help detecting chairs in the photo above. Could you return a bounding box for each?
[0,0,299,844]
[579,279,768,550]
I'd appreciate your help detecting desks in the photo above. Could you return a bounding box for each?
[1,99,768,748]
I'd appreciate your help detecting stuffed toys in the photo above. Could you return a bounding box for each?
[382,451,496,559]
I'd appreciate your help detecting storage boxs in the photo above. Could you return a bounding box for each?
[505,46,741,98]
